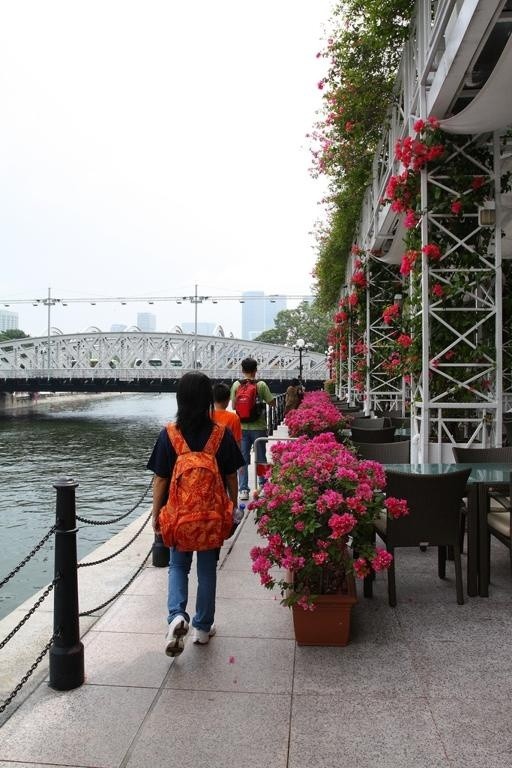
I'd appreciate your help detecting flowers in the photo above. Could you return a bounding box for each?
[247,433,410,612]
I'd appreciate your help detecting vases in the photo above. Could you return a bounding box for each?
[277,544,358,646]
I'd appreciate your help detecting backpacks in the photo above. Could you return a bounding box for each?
[235,378,259,423]
[159,423,234,552]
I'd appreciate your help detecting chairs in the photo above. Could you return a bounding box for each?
[352,426,396,442]
[348,440,411,558]
[452,447,512,555]
[364,467,472,606]
[324,393,367,417]
[351,417,392,430]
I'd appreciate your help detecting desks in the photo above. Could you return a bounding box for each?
[352,462,512,597]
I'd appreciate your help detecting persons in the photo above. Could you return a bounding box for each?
[230,357,276,500]
[284,378,301,412]
[208,382,242,449]
[146,371,246,658]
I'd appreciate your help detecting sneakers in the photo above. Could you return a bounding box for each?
[166,616,189,657]
[239,490,249,500]
[192,624,215,644]
